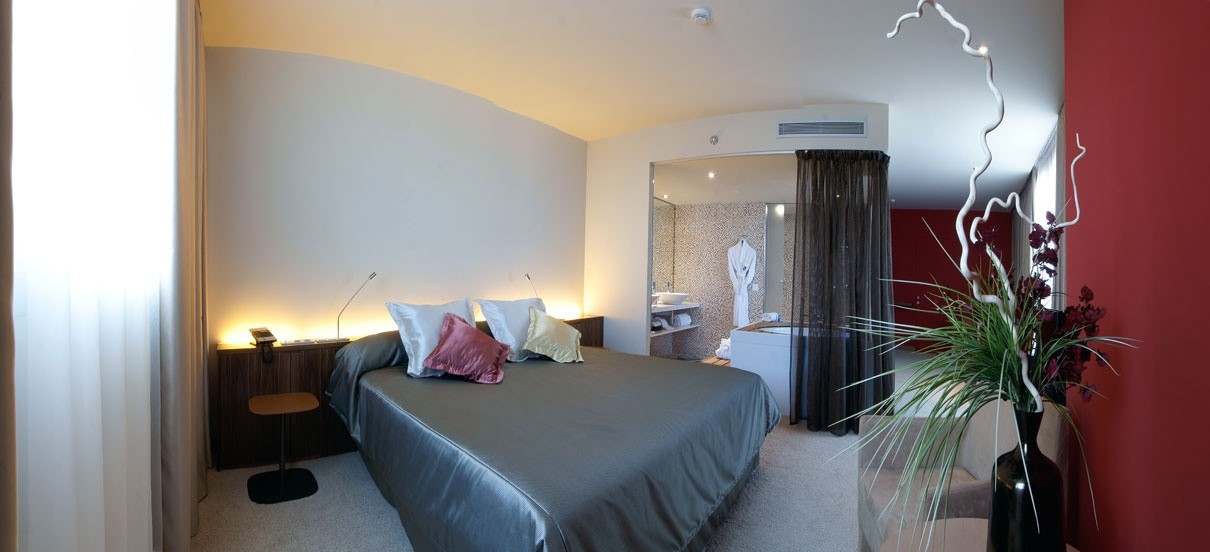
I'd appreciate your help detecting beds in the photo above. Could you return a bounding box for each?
[325,321,782,552]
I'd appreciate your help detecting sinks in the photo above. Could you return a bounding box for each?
[652,295,659,304]
[656,293,689,305]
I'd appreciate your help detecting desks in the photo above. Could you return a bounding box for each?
[246,389,321,505]
[880,517,1082,552]
[214,337,361,472]
[565,312,604,348]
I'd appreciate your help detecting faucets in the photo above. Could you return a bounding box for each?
[652,281,657,293]
[667,281,672,293]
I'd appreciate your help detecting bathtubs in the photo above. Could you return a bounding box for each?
[758,326,851,338]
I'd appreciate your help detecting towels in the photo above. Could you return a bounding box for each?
[673,313,692,327]
[762,312,779,322]
[715,337,730,360]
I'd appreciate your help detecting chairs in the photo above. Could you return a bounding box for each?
[858,389,1062,552]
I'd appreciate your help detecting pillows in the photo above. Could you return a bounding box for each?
[521,305,585,365]
[475,297,548,363]
[422,312,511,385]
[384,296,477,379]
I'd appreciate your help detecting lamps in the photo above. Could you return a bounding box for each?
[337,271,377,341]
[525,273,539,298]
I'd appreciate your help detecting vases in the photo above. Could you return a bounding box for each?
[985,402,1067,552]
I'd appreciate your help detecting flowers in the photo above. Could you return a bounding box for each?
[836,213,1111,552]
